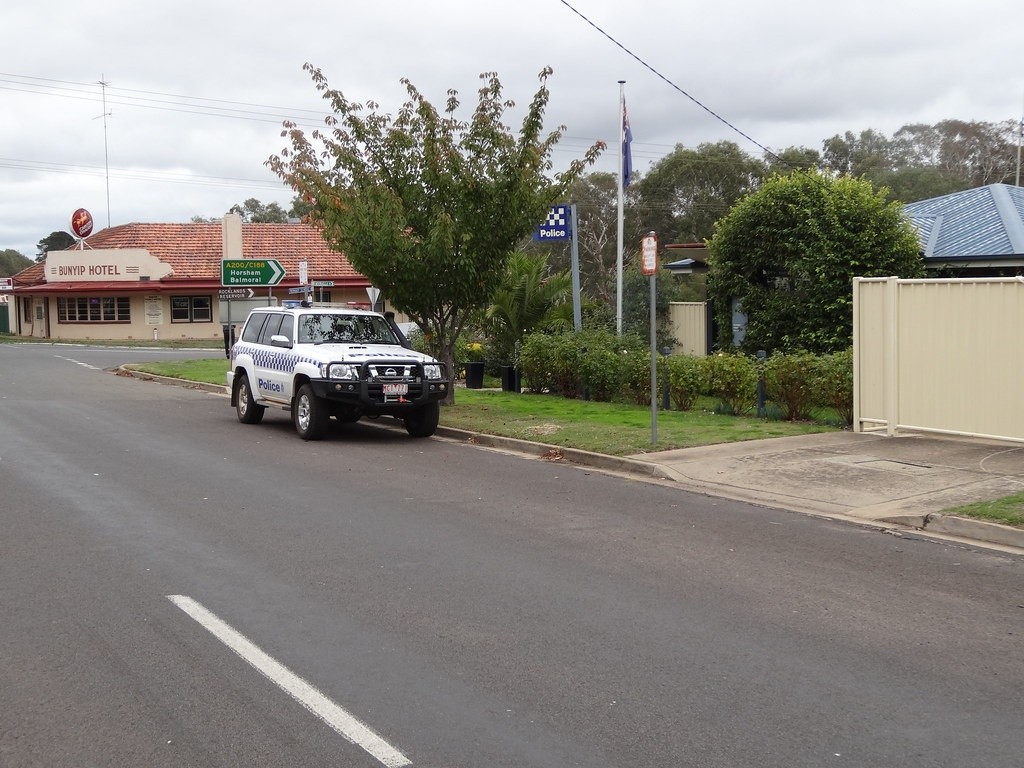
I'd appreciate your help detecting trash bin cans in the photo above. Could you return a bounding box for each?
[501,366,515,392]
[223,325,236,359]
[466,362,484,389]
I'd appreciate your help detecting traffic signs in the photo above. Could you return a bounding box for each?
[222,258,287,287]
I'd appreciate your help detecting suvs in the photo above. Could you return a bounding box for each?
[224,300,450,442]
